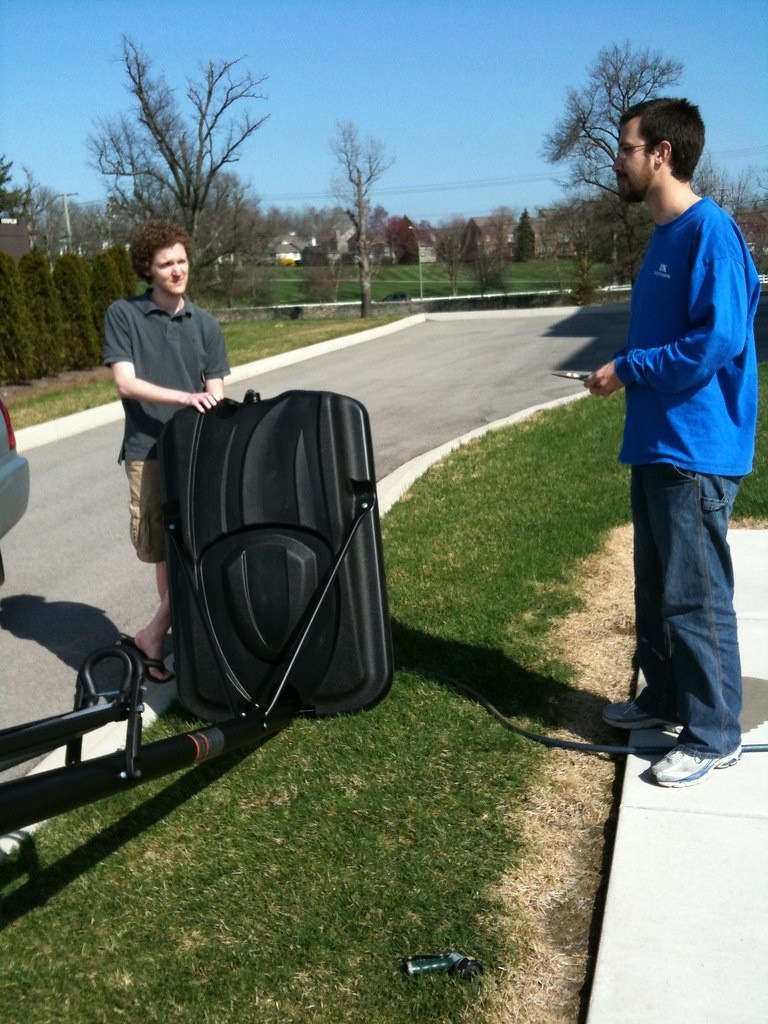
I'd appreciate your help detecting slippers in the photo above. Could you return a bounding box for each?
[125,640,175,682]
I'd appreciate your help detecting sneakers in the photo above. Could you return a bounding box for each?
[650,743,745,787]
[601,699,682,729]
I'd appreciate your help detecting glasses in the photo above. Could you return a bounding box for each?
[618,142,655,157]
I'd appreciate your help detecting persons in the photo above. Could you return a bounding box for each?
[101,222,230,683]
[583,99,763,791]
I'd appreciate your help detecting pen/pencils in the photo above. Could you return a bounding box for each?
[550,372,590,381]
